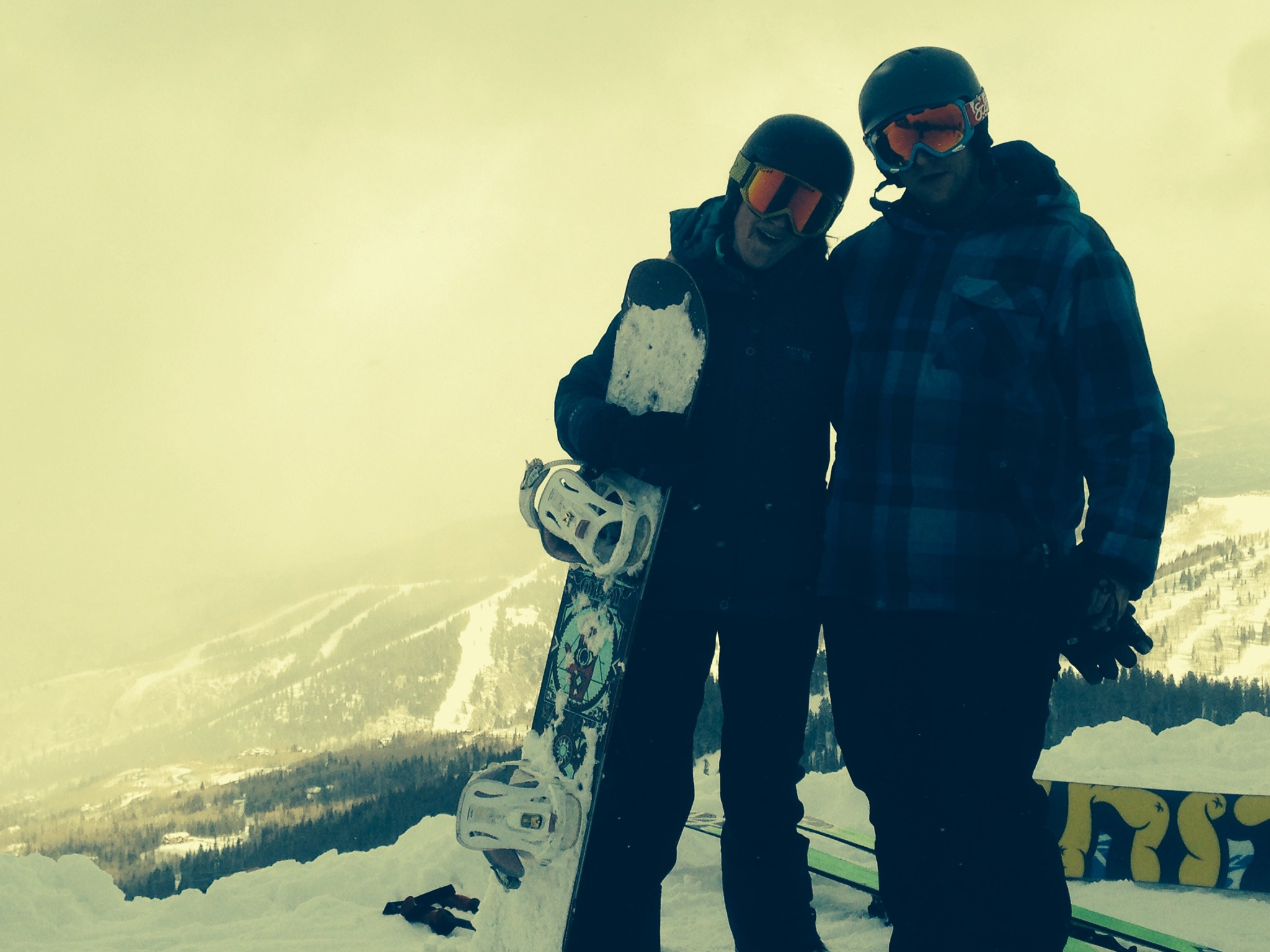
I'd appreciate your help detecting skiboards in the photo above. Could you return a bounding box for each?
[682,810,1224,952]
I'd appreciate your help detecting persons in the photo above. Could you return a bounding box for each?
[825,47,1173,952]
[555,115,852,952]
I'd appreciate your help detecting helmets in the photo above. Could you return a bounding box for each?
[862,46,986,183]
[725,114,856,240]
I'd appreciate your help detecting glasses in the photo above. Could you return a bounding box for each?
[865,97,974,171]
[738,165,843,240]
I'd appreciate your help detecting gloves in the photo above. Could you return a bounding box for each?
[569,403,695,487]
[1053,547,1154,687]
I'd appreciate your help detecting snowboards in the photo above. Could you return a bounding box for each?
[1033,777,1270,893]
[453,255,710,952]
